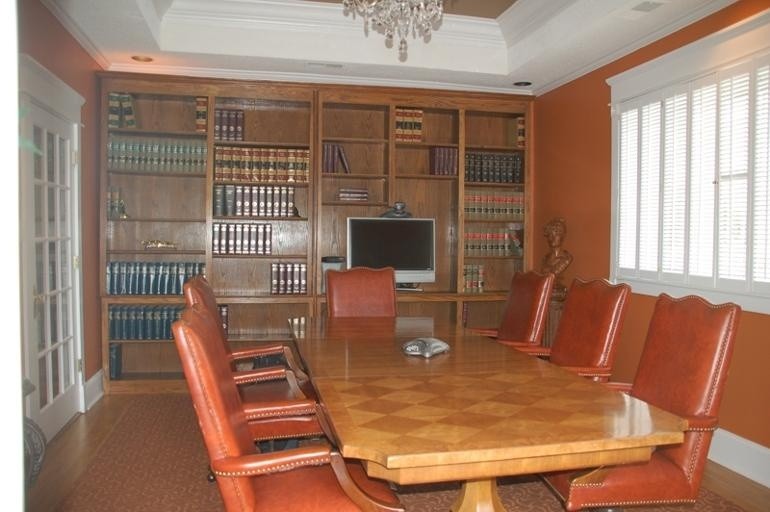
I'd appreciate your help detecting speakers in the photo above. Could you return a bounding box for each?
[321,256,346,294]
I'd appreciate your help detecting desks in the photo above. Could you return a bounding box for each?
[287,314,692,511]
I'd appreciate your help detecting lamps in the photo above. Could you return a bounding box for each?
[341,0,446,63]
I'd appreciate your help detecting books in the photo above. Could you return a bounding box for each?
[210,220,273,256]
[213,107,245,142]
[463,149,527,258]
[106,92,120,130]
[509,115,526,149]
[269,260,307,296]
[109,344,124,380]
[211,145,312,217]
[110,186,120,219]
[105,140,207,177]
[431,145,458,176]
[322,142,351,175]
[220,305,229,342]
[120,94,138,129]
[394,107,425,143]
[105,261,206,341]
[106,221,115,252]
[337,187,370,202]
[194,97,207,133]
[463,302,472,327]
[286,313,306,340]
[462,264,487,294]
[105,185,111,218]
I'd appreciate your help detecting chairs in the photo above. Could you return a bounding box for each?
[524,271,635,385]
[464,268,556,360]
[533,286,740,512]
[323,268,401,318]
[169,270,412,511]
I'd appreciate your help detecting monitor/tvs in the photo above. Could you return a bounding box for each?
[347,216,435,292]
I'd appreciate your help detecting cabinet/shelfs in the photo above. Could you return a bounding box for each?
[96,69,539,399]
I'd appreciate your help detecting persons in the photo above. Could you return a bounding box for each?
[538,219,577,273]
